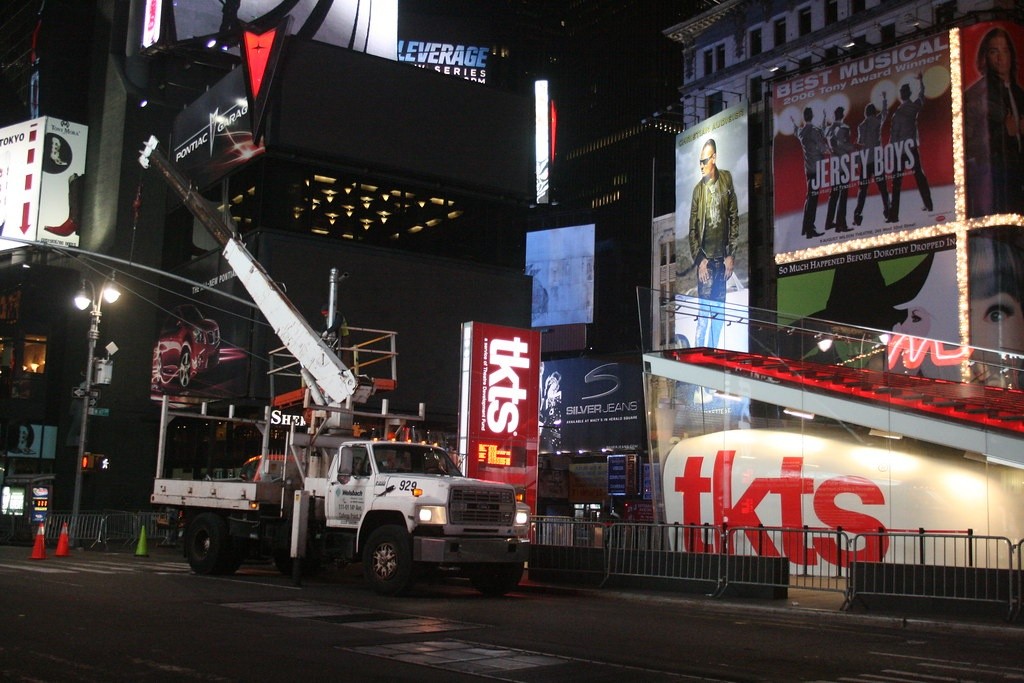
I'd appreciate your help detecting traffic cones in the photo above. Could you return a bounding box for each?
[55,523,71,557]
[135,524,152,559]
[26,523,48,561]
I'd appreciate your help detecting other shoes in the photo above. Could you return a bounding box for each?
[835,227,854,233]
[826,223,836,230]
[922,206,933,211]
[885,218,898,223]
[853,221,861,226]
[806,230,825,239]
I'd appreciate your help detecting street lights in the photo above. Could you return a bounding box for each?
[67,271,123,550]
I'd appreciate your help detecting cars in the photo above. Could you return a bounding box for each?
[152,302,222,389]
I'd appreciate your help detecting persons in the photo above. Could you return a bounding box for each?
[574,508,622,549]
[320,304,349,369]
[967,235,1024,391]
[688,137,739,347]
[961,28,1024,218]
[793,72,933,240]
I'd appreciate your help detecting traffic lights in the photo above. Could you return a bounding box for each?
[96,454,111,470]
[81,453,95,470]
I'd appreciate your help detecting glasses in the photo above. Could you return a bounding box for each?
[700,152,714,166]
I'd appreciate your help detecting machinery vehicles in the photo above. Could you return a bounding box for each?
[138,135,532,598]
